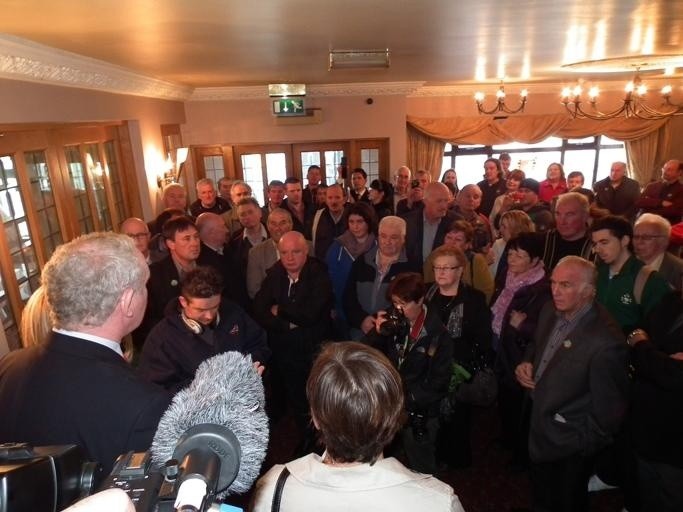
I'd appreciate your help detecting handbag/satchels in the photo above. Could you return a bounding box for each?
[458,341,514,406]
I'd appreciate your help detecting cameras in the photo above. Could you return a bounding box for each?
[411,179,419,189]
[380,311,409,340]
[514,192,525,200]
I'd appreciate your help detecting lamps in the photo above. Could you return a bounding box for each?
[472,80,528,119]
[559,64,683,123]
[156,146,188,187]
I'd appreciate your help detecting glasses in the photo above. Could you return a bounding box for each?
[632,235,663,241]
[432,266,459,272]
[128,234,148,240]
